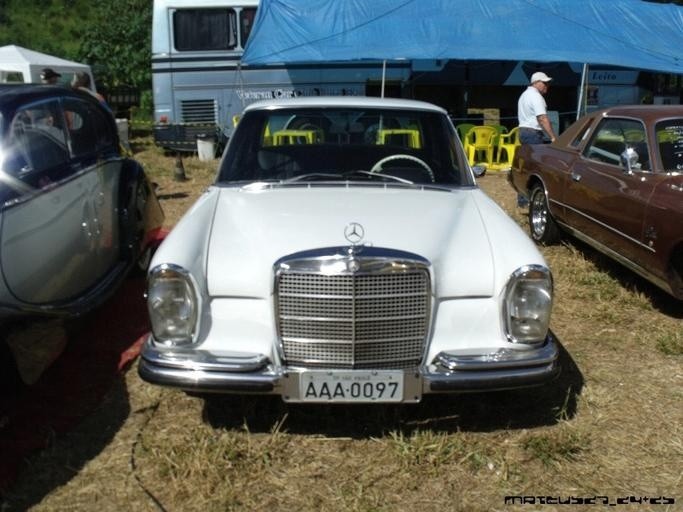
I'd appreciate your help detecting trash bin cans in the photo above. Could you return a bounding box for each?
[195,131,216,162]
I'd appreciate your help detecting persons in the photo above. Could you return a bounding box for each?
[517,72,557,216]
[27,67,70,144]
[63,71,114,165]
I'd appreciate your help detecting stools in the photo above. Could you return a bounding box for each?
[373,130,420,148]
[271,129,316,146]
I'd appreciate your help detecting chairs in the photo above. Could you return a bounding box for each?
[252,142,428,180]
[454,123,519,172]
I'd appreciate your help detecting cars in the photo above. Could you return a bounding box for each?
[510,104,682,307]
[138,93,563,405]
[0,82,165,333]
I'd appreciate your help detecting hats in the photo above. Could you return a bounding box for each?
[530,72,554,85]
